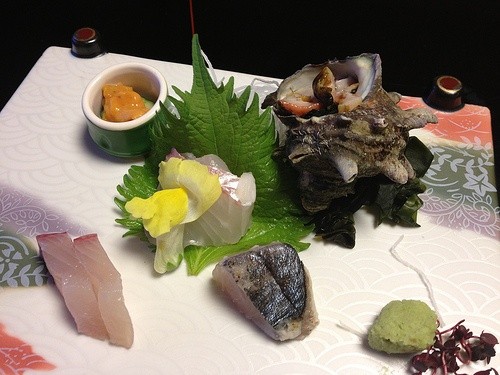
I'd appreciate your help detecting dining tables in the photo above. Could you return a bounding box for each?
[0,46,500,375]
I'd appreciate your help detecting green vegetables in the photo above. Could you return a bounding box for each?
[114,33,316,278]
